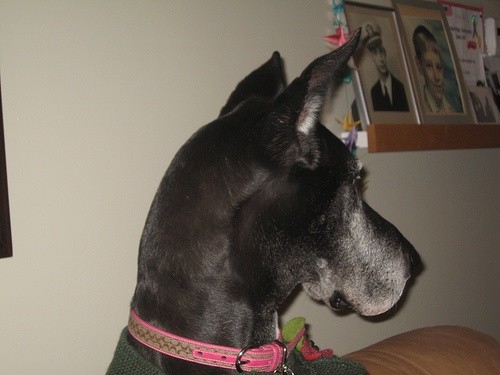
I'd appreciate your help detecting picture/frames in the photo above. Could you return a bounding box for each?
[341,0,421,129]
[391,0,478,129]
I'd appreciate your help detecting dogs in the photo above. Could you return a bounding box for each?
[104,27,423,375]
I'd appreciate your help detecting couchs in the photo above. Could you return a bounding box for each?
[340,324,500,375]
